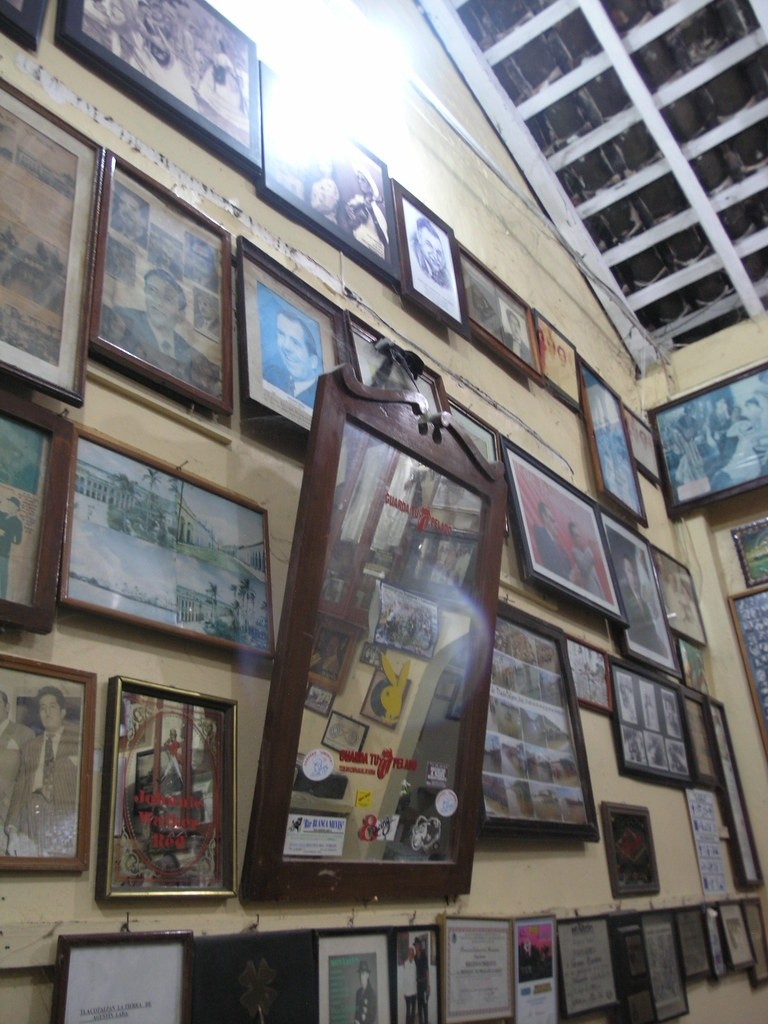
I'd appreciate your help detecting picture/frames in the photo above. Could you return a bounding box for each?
[0,0,768,1024]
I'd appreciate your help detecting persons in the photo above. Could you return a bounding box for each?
[533,502,572,582]
[264,311,318,409]
[403,937,431,1024]
[574,647,585,673]
[631,420,658,478]
[345,165,390,260]
[353,959,377,1024]
[570,521,606,602]
[557,346,578,403]
[4,686,79,858]
[665,571,687,621]
[0,690,34,856]
[100,194,220,399]
[680,578,700,627]
[595,656,605,681]
[667,370,768,492]
[0,496,23,600]
[83,0,247,127]
[621,551,663,656]
[504,309,535,368]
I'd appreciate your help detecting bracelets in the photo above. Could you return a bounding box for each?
[373,220,377,225]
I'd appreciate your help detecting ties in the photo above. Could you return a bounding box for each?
[44,734,56,782]
